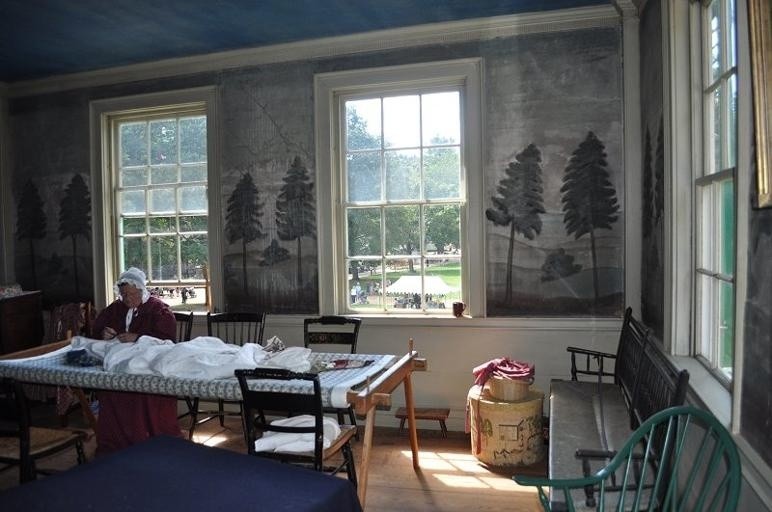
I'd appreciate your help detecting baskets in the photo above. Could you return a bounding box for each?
[488,371,534,401]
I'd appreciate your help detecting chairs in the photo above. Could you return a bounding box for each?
[288,316,361,443]
[0,377,87,485]
[234,367,358,490]
[173,312,192,424]
[189,309,266,441]
[511,407,740,512]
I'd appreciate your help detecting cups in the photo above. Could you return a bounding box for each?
[452,301,467,318]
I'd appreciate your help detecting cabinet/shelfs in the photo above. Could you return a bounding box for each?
[0,290,45,422]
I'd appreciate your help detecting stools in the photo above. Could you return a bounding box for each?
[395,406,449,437]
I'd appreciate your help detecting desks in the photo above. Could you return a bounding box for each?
[0,432,363,512]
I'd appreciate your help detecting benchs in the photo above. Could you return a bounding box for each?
[547,308,688,512]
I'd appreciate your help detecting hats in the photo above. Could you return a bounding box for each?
[114,267,151,304]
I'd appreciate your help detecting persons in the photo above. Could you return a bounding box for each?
[148,286,197,304]
[89,267,183,446]
[348,279,445,308]
